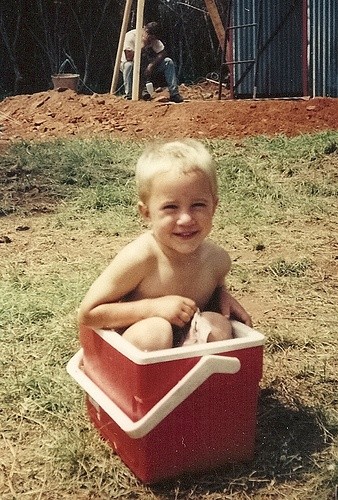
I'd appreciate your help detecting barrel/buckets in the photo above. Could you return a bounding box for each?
[51,60,80,95]
[66,309,266,484]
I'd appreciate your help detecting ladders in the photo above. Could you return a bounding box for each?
[217,0,257,100]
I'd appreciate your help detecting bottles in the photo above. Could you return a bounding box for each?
[146,81,155,95]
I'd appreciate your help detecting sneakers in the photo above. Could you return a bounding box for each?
[170,94,183,103]
[126,93,132,100]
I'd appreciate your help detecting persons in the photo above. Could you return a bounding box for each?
[78,138,252,353]
[119,22,184,103]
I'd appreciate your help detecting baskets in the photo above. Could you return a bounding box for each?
[51,60,80,94]
